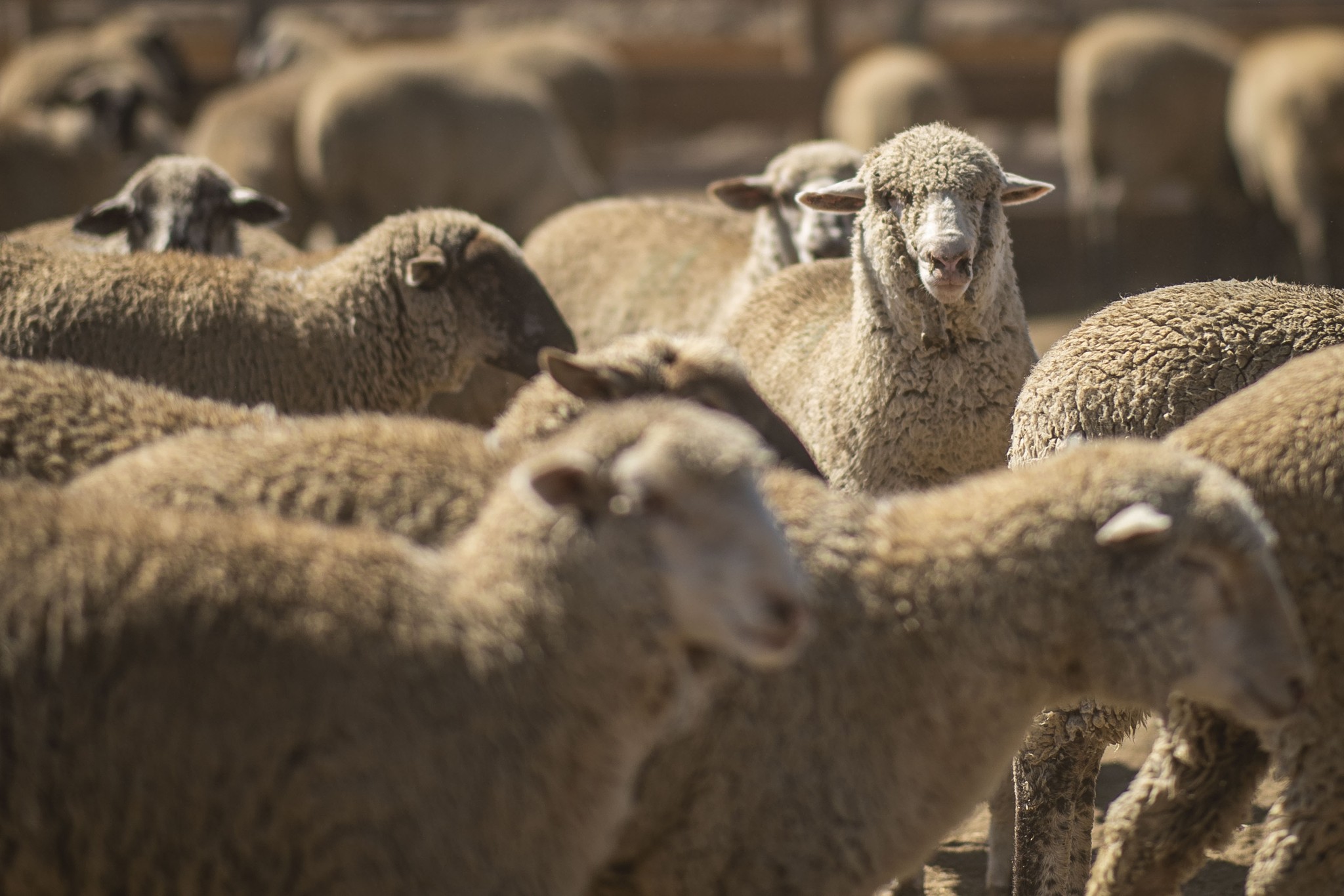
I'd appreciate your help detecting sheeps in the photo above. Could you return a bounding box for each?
[0,2,1343,896]
